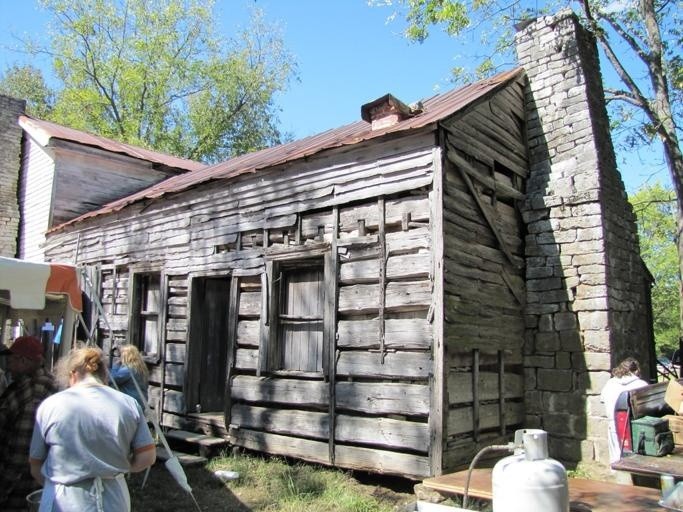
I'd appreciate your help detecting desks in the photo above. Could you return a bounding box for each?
[611,448,683,489]
[423,467,683,512]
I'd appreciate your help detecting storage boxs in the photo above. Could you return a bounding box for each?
[664,378,683,414]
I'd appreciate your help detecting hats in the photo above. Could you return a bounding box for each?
[0,336,41,361]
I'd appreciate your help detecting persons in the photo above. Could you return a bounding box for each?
[671,337,682,377]
[0,333,157,512]
[597,356,651,484]
